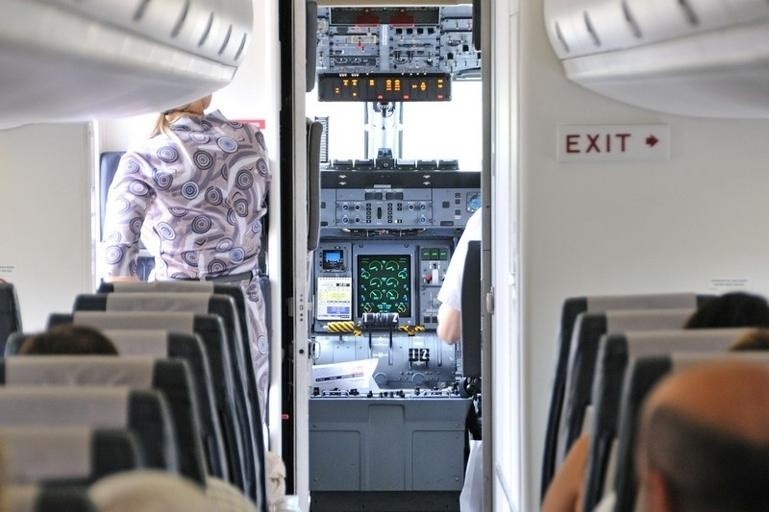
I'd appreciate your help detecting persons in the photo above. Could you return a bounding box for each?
[434,206,483,344]
[631,350,768,511]
[592,331,769,512]
[540,292,767,512]
[103,93,269,450]
[15,325,121,354]
